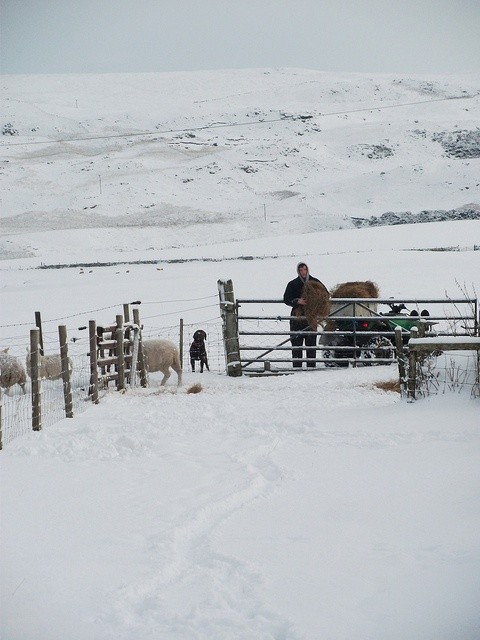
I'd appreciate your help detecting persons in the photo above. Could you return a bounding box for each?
[282,261,330,367]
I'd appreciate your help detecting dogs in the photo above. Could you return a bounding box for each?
[189,330,210,373]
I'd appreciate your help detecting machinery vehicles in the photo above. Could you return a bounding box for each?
[319,297,440,368]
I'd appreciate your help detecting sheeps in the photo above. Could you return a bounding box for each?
[104,334,182,386]
[26,347,73,381]
[0,347,27,394]
